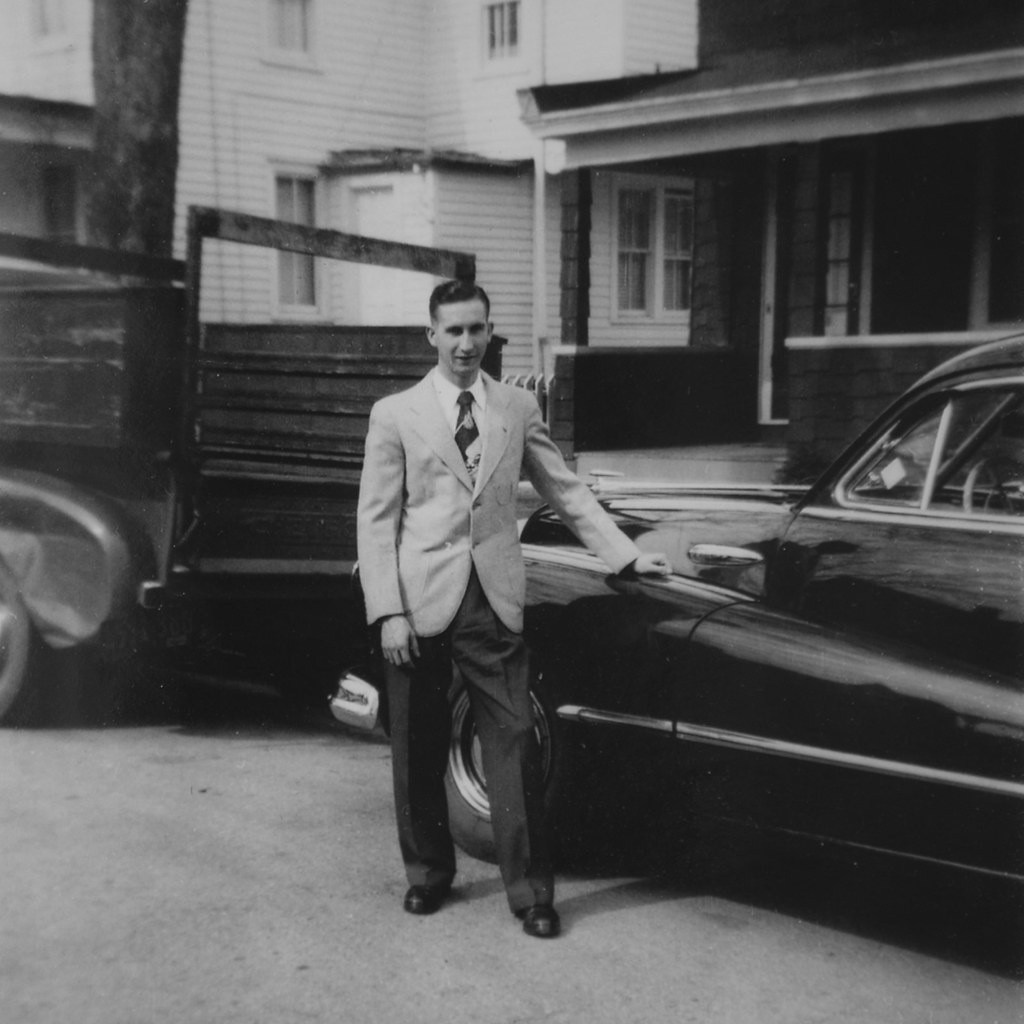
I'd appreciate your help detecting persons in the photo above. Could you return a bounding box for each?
[356,281,674,938]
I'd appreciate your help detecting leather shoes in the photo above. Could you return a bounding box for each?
[516,904,561,935]
[404,884,452,913]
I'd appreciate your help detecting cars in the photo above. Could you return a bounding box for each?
[327,332,1023,927]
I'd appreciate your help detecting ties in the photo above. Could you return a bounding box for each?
[454,391,482,488]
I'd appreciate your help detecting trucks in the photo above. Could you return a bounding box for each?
[0,206,478,721]
